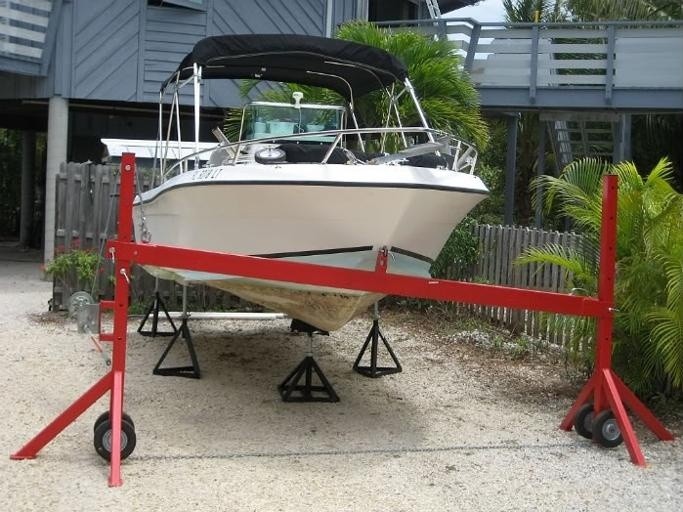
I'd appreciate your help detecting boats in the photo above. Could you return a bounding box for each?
[131,32,489,336]
